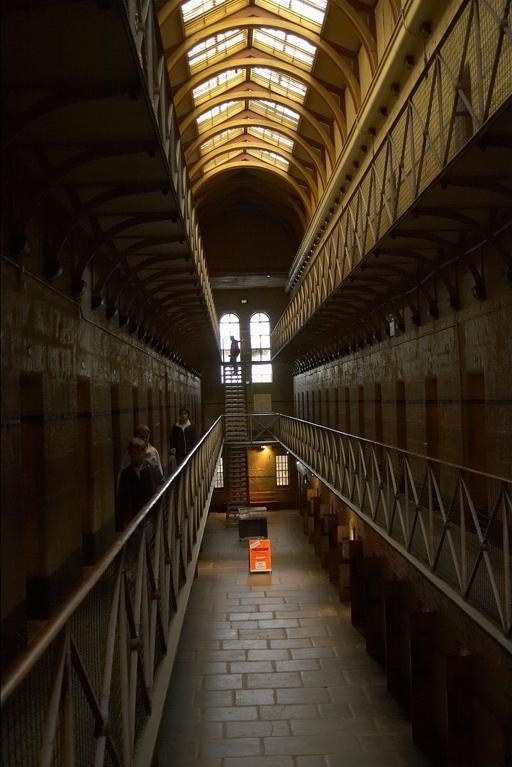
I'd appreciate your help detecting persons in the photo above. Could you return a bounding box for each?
[169,406,197,476]
[117,422,164,494]
[228,335,241,376]
[115,436,166,535]
